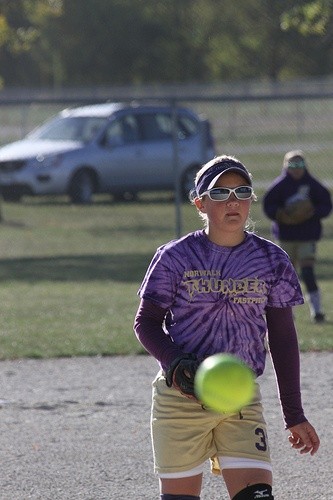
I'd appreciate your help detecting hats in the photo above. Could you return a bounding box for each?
[195,160,253,196]
[283,151,306,163]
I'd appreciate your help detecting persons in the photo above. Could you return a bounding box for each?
[262,154,331,323]
[133,154,321,500]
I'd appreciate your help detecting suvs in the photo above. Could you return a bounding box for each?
[0,100,216,206]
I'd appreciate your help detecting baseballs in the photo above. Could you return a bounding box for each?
[194,354,256,411]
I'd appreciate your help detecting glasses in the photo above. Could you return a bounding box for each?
[199,185,253,201]
[285,161,305,169]
